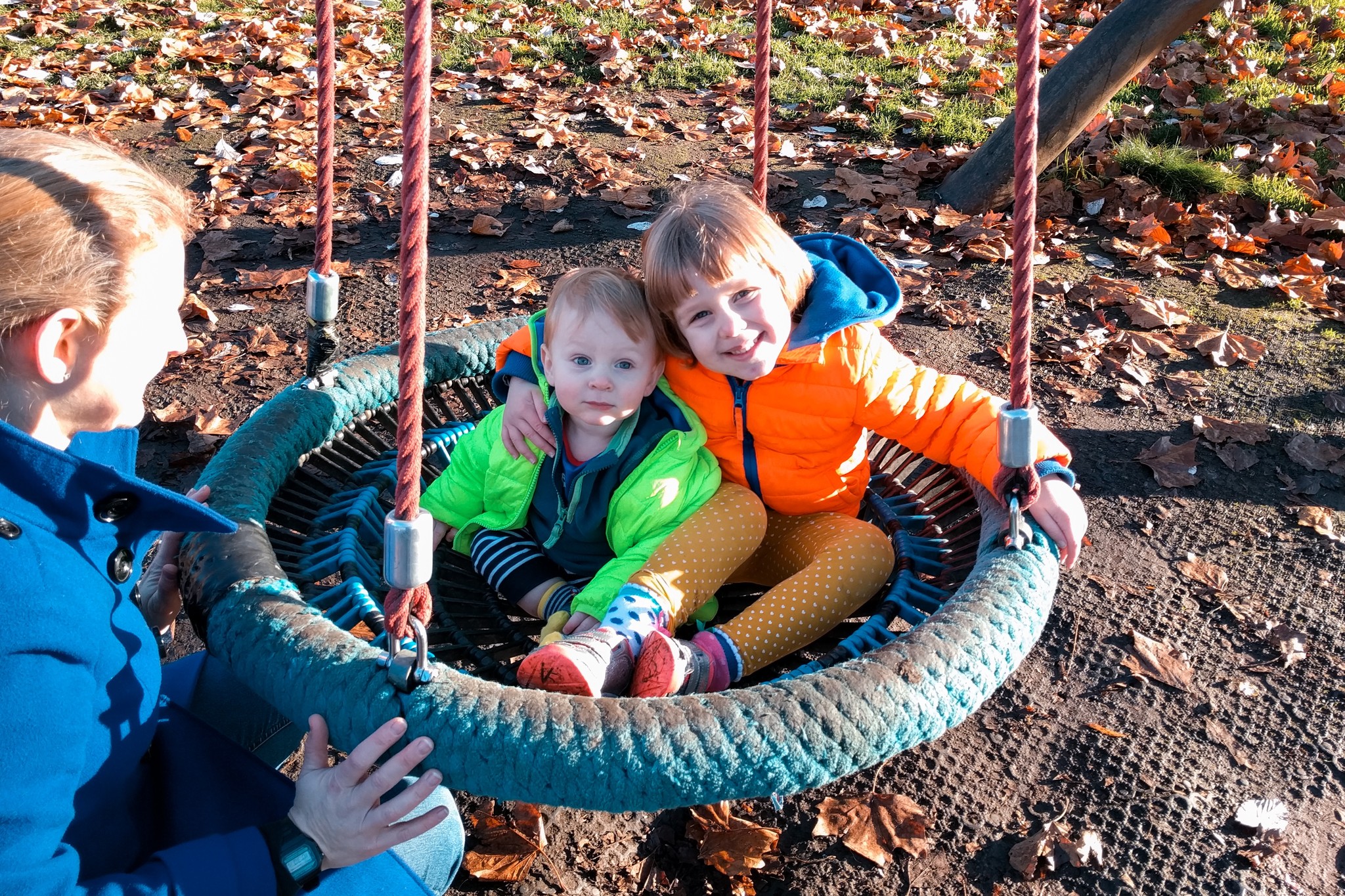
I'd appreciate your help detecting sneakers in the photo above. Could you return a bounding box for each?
[515,626,634,698]
[631,630,694,697]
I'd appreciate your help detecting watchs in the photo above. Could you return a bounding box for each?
[257,811,325,893]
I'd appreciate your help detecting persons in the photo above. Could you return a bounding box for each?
[1,127,466,896]
[493,178,1090,699]
[420,267,725,650]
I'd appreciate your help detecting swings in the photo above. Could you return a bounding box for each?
[183,1,1061,818]
